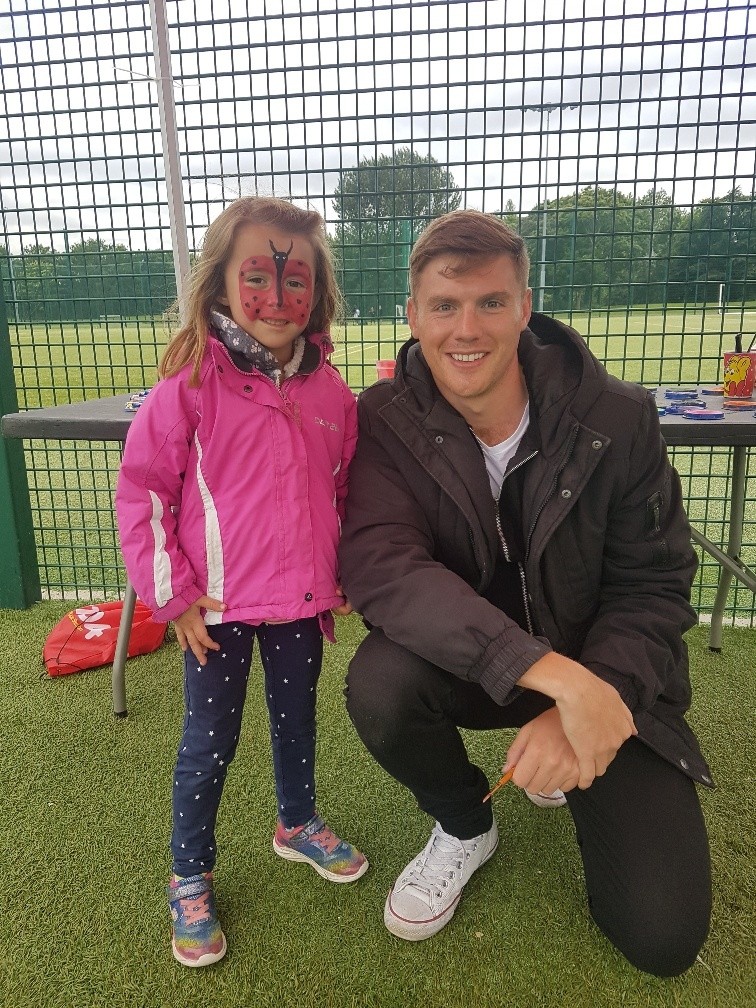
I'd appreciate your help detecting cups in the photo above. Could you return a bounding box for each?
[723,350,756,410]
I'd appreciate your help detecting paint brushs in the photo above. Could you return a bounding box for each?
[482,767,515,803]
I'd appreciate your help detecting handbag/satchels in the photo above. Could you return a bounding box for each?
[43,599,167,676]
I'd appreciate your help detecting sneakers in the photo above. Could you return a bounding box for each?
[272,809,371,882]
[524,781,566,809]
[384,806,499,942]
[167,869,227,968]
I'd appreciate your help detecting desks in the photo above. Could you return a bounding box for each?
[1,388,756,717]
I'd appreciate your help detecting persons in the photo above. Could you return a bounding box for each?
[114,194,370,967]
[335,209,716,978]
[350,304,379,326]
